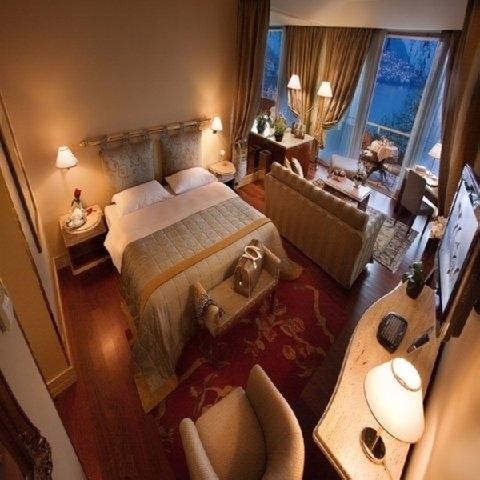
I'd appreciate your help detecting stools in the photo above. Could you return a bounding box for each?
[328,154,358,180]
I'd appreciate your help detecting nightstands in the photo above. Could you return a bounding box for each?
[59,205,111,276]
[209,159,236,191]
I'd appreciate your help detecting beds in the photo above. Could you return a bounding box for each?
[78,118,305,413]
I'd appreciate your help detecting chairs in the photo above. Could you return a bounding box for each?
[393,170,434,238]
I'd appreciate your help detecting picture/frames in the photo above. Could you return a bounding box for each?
[1,95,42,253]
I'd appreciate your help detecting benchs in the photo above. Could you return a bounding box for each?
[194,243,281,368]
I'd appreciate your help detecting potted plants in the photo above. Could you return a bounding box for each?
[401,261,424,299]
[352,171,363,189]
[256,111,267,134]
[273,116,286,141]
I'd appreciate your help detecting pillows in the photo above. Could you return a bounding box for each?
[284,156,291,170]
[110,180,174,218]
[291,156,303,177]
[165,167,219,193]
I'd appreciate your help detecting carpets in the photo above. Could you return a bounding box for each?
[124,271,355,480]
[311,177,419,274]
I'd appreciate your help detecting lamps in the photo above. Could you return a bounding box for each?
[317,81,333,98]
[359,357,426,463]
[425,142,443,187]
[210,116,223,134]
[54,145,77,169]
[287,74,301,90]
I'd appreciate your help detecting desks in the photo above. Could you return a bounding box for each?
[313,278,445,480]
[246,116,316,181]
[394,168,439,214]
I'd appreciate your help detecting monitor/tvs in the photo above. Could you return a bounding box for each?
[434,164,480,338]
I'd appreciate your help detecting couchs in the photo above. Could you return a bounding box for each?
[265,162,385,287]
[180,365,307,480]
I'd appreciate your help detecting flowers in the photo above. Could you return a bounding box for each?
[70,187,84,206]
